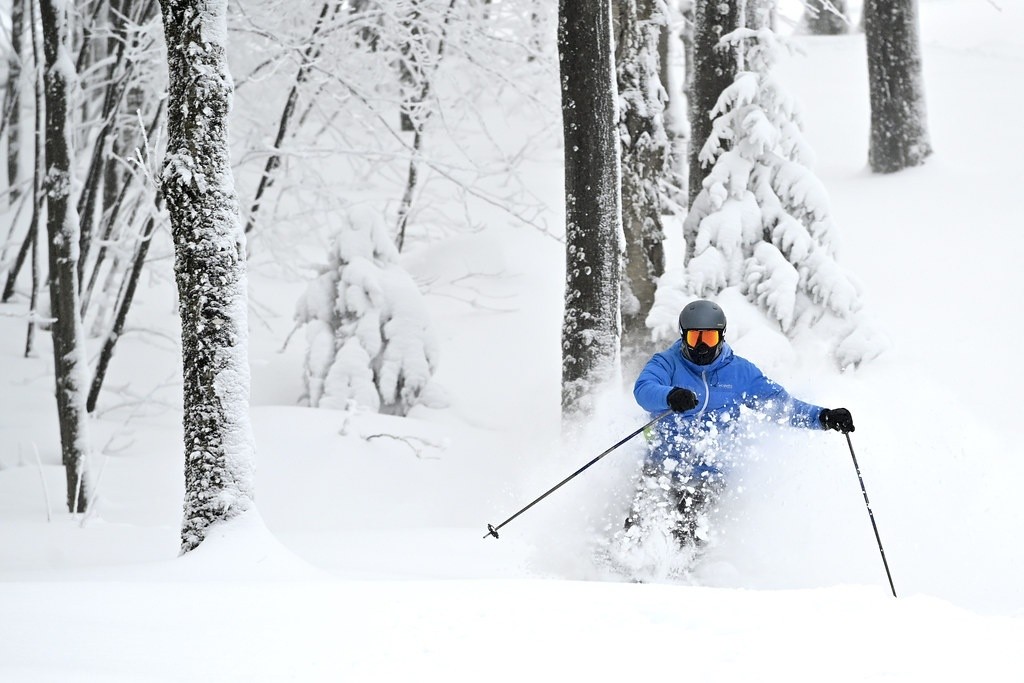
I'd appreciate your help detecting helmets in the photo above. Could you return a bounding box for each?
[679,300,726,366]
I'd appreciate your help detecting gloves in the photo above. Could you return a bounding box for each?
[666,388,699,413]
[819,408,855,434]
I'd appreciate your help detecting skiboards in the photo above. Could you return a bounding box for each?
[597,536,707,586]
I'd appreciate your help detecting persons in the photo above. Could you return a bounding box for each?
[624,300,854,548]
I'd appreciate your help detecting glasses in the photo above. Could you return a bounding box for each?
[686,330,719,347]
